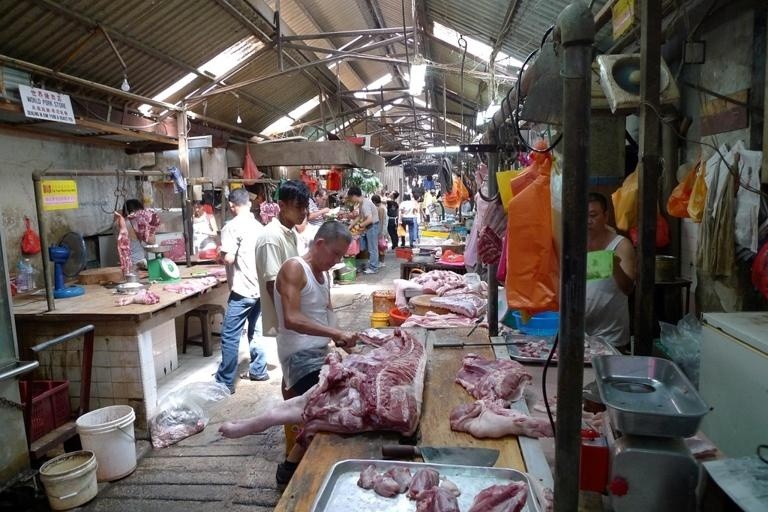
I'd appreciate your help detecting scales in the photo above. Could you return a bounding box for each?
[143,244,182,282]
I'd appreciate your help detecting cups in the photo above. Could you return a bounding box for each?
[655,255,676,284]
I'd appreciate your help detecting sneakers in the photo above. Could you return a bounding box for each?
[275,462,298,491]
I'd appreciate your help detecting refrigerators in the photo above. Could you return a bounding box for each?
[698,310,768,459]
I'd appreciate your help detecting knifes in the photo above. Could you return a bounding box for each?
[381,443,500,467]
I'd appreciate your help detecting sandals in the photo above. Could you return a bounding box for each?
[240,372,251,379]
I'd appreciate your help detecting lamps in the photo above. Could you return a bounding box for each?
[425,111,446,155]
[119,69,132,95]
[483,57,504,124]
[446,92,464,156]
[402,0,431,99]
[234,94,244,126]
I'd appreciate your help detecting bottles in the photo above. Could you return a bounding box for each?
[16,260,28,293]
[25,258,34,290]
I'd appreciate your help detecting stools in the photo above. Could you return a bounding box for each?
[181,301,227,358]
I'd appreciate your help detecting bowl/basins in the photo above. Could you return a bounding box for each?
[388,307,412,326]
[584,248,615,280]
[511,310,559,337]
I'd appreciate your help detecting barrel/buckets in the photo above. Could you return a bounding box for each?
[76,405,138,482]
[338,255,356,281]
[395,245,412,260]
[390,306,413,325]
[40,450,99,509]
[655,254,677,281]
[371,311,389,328]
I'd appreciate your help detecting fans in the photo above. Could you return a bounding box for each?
[47,229,91,301]
[596,49,684,116]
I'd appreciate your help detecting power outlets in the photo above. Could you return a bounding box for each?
[474,86,488,128]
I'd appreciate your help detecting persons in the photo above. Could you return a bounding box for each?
[582,193,637,351]
[425,189,437,222]
[214,187,265,394]
[307,188,331,226]
[413,192,421,245]
[399,191,418,248]
[381,190,400,250]
[371,194,388,267]
[191,196,218,254]
[272,220,359,487]
[253,180,313,461]
[347,187,380,274]
[122,199,156,264]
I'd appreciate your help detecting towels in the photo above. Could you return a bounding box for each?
[694,153,714,275]
[706,153,736,280]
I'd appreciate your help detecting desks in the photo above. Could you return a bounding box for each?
[397,249,468,280]
[270,314,551,511]
[631,277,692,332]
[12,250,234,441]
